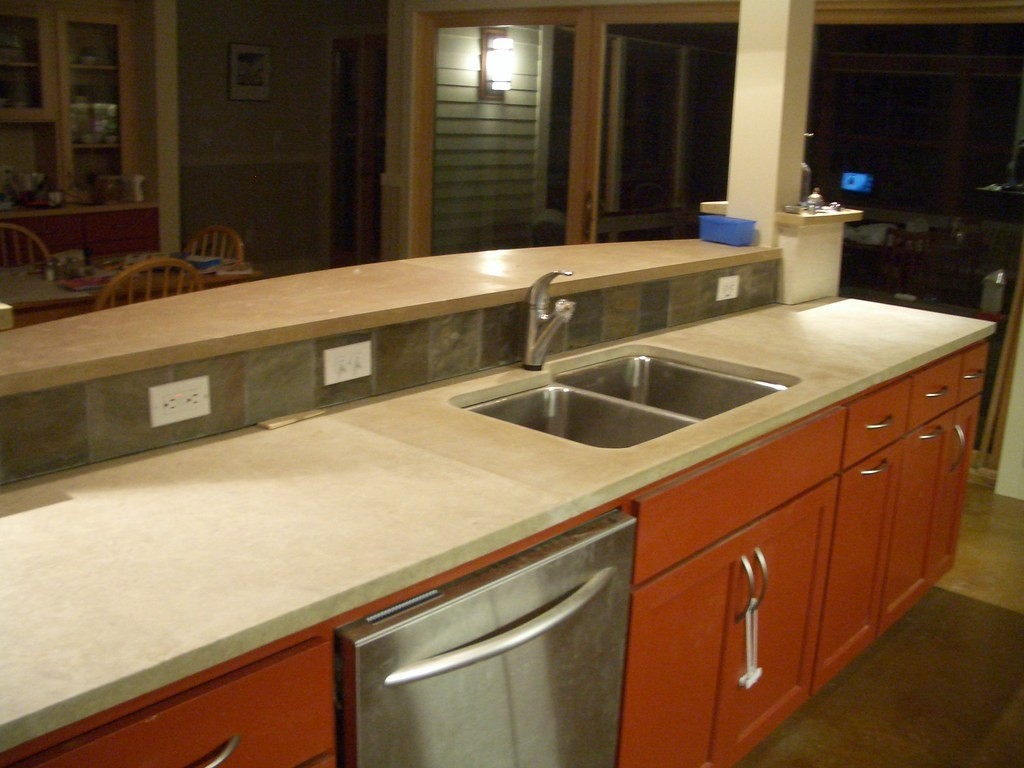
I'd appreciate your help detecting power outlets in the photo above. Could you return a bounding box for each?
[716,275,738,300]
[325,341,372,385]
[148,375,211,428]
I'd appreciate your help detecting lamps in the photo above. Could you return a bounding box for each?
[484,35,515,90]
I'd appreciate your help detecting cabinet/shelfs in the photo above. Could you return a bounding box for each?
[616,340,988,768]
[7,634,338,768]
[0,6,159,266]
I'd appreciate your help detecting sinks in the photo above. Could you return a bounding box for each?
[552,354,786,420]
[465,383,698,449]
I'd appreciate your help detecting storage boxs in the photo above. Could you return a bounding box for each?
[698,214,755,246]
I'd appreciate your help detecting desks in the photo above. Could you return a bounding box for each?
[0,250,263,328]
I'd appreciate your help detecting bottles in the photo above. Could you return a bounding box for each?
[876,226,939,303]
[44,259,56,282]
[68,257,80,279]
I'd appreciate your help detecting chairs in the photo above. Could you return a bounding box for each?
[0,222,50,267]
[182,225,244,261]
[95,258,206,313]
[877,228,938,295]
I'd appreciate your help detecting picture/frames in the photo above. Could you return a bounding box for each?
[229,43,273,101]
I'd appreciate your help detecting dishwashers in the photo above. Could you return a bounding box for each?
[336,500,638,768]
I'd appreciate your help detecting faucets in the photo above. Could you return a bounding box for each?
[521,269,576,372]
